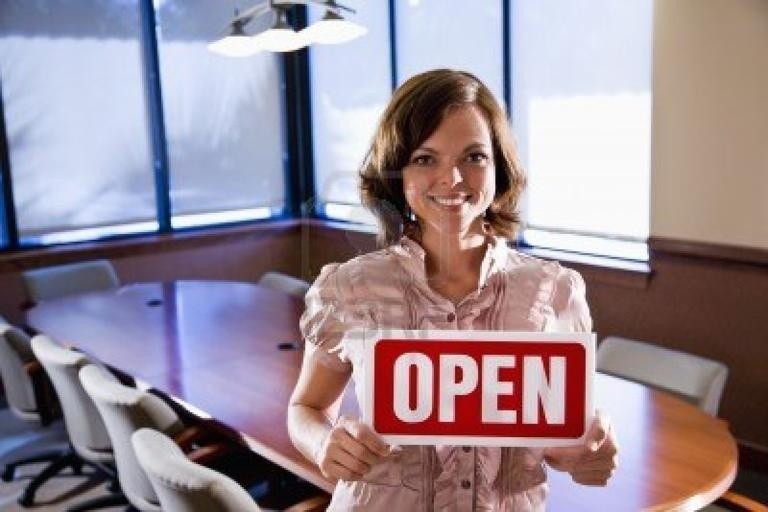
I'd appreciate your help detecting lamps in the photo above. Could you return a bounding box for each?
[205,0,368,57]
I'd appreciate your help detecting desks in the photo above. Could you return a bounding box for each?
[24,280,740,512]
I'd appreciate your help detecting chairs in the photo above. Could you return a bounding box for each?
[597,337,728,417]
[0,317,118,508]
[32,334,121,512]
[19,260,121,309]
[77,365,209,512]
[259,272,311,299]
[130,427,331,512]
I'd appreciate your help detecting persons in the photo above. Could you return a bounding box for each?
[286,68,623,511]
